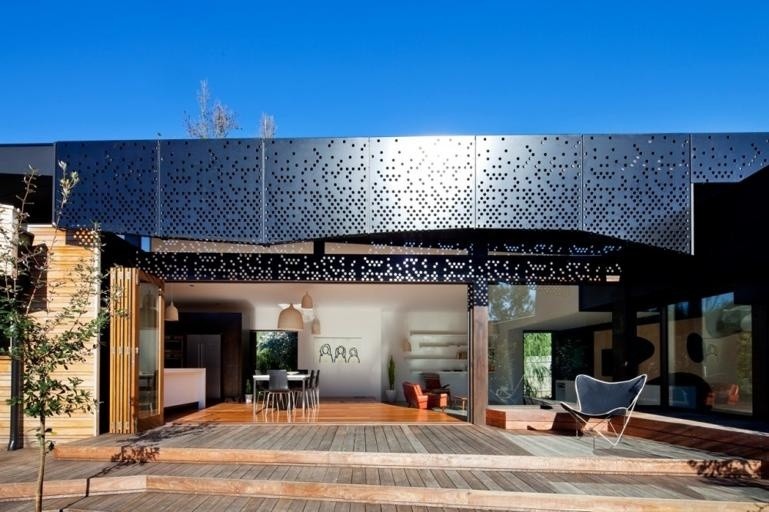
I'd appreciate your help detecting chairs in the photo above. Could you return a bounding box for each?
[560,374,648,450]
[403,381,447,412]
[422,373,451,409]
[252,369,320,416]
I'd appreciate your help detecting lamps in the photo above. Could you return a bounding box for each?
[164,283,178,321]
[278,283,321,335]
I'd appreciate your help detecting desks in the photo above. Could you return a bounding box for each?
[453,395,468,410]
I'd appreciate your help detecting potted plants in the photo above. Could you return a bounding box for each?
[385,355,398,403]
[244,379,252,404]
